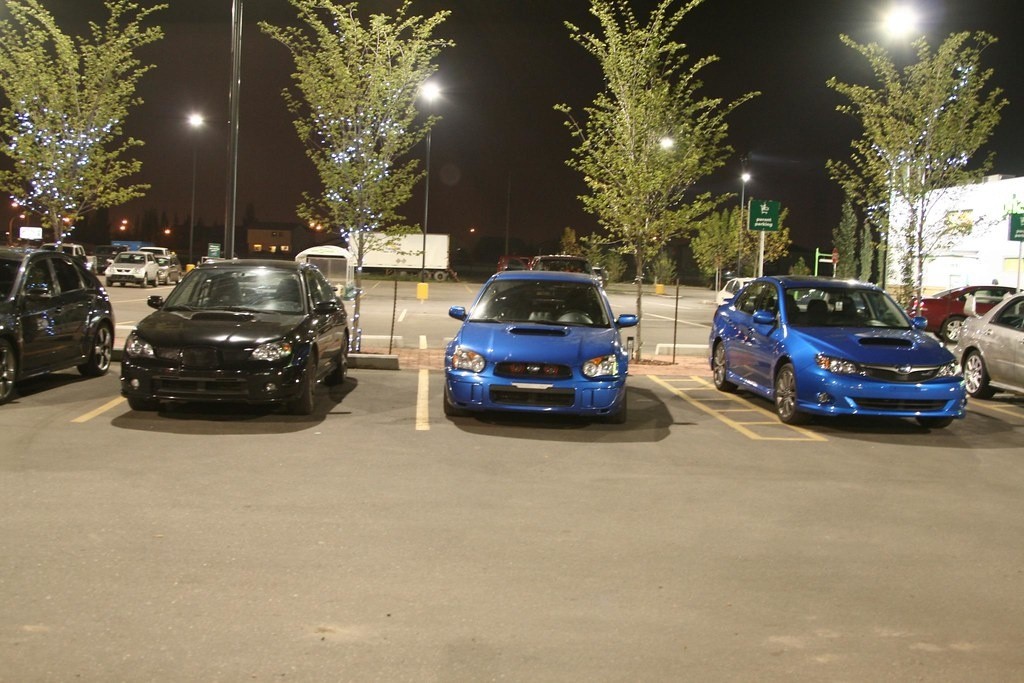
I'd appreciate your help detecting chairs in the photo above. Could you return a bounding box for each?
[275,279,301,307]
[773,295,800,323]
[557,292,598,323]
[807,300,828,314]
[207,277,244,307]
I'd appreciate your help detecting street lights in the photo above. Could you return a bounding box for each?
[188,115,203,263]
[645,137,673,230]
[737,172,751,278]
[417,82,440,281]
[8,213,26,244]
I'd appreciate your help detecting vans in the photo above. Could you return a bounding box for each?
[139,247,168,256]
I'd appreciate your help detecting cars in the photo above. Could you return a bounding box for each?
[717,277,756,306]
[953,291,1024,399]
[907,285,1024,344]
[497,254,533,271]
[89,244,131,274]
[502,254,593,276]
[593,269,609,289]
[120,259,350,415]
[105,252,160,288]
[444,271,639,424]
[708,275,968,429]
[156,254,182,285]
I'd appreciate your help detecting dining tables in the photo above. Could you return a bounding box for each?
[497,292,531,320]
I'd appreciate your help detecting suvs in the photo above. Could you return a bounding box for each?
[0,248,114,405]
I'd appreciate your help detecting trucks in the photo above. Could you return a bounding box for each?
[40,244,97,277]
